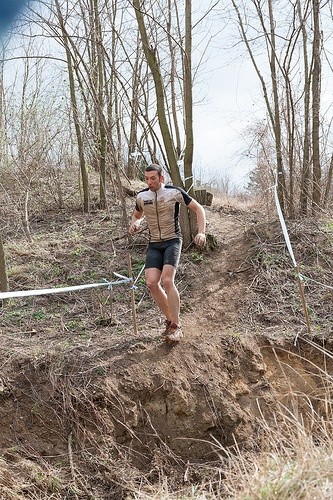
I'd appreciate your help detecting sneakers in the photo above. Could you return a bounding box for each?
[161,320,172,336]
[166,323,183,341]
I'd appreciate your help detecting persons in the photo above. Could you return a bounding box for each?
[130,164,205,340]
